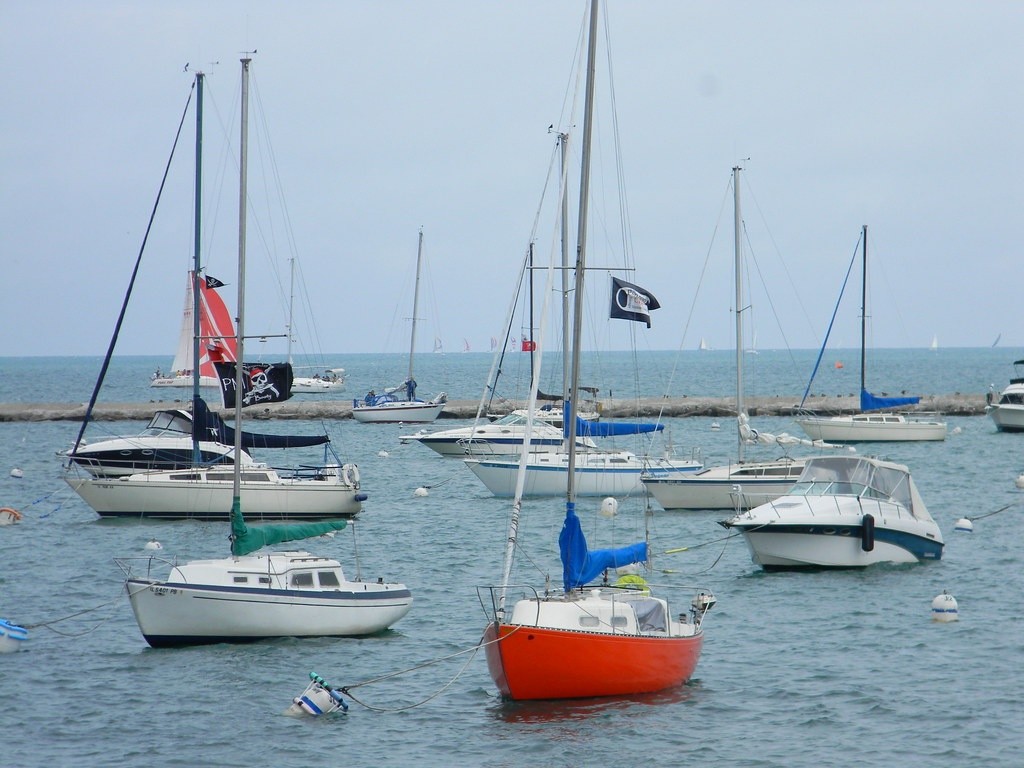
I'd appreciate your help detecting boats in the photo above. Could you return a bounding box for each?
[718,452,945,573]
[982,358,1024,431]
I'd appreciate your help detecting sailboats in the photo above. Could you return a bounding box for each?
[111,51,418,649]
[638,159,806,519]
[792,224,952,442]
[59,49,840,520]
[473,1,721,700]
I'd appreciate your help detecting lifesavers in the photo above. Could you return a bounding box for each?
[615,576,650,592]
[342,463,360,486]
